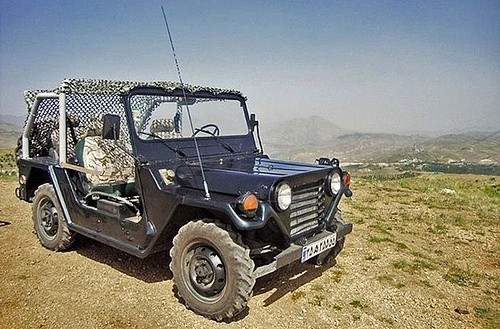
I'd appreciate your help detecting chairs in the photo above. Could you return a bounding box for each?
[147,117,186,140]
[74,112,139,200]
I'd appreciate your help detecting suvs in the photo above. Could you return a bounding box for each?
[12,79,355,328]
